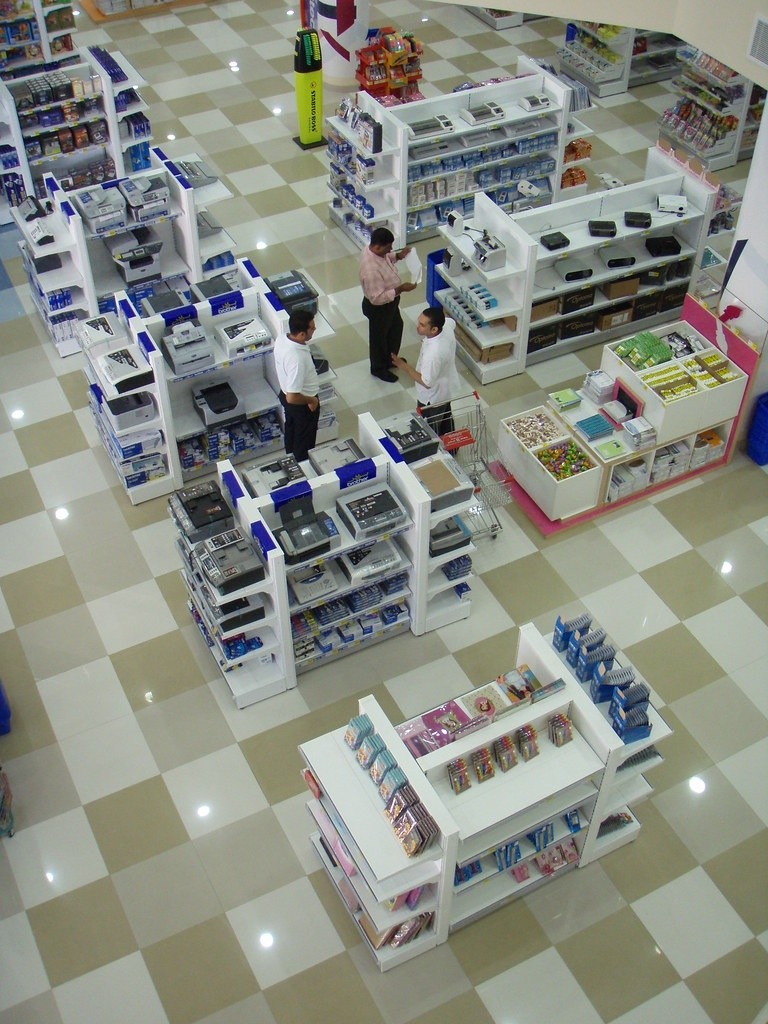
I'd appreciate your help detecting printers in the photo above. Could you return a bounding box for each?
[17,160,475,672]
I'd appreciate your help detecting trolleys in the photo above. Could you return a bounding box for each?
[419,392,515,542]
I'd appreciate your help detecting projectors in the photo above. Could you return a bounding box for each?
[541,231,571,251]
[553,257,594,282]
[589,221,616,238]
[598,245,637,269]
[655,194,687,214]
[645,236,682,257]
[625,212,651,228]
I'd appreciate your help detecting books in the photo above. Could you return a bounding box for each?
[548,369,657,460]
[609,457,650,500]
[692,430,724,465]
[651,440,691,484]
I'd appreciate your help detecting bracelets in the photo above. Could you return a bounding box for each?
[396,252,405,260]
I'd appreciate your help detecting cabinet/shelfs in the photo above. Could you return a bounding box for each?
[167,411,479,710]
[74,256,341,506]
[496,319,750,523]
[434,144,743,386]
[0,45,155,226]
[8,145,238,360]
[0,0,81,73]
[737,102,766,163]
[628,31,682,88]
[555,20,637,97]
[656,60,755,172]
[297,622,676,975]
[324,55,598,251]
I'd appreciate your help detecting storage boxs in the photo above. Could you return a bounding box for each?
[0,0,690,666]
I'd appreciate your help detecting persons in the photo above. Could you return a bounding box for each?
[359,228,417,382]
[391,307,462,457]
[273,310,321,462]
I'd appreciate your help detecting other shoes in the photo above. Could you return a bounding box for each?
[371,357,407,382]
